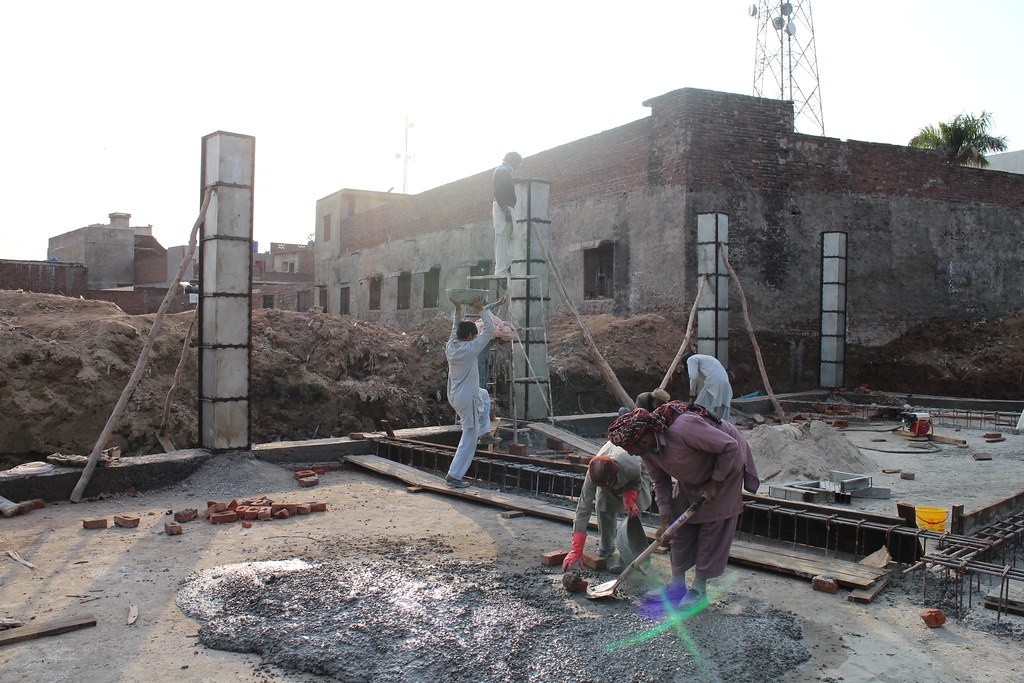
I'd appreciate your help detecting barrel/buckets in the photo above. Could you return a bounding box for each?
[915,505,948,534]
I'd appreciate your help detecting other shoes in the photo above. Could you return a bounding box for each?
[594,545,615,559]
[610,557,625,572]
[679,587,706,611]
[645,583,687,603]
[446,481,470,487]
[478,437,503,445]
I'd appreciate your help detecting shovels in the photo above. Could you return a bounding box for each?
[584,496,708,600]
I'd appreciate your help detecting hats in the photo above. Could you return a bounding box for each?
[651,388,670,401]
[589,456,618,486]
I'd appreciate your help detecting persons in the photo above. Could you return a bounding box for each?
[682,353,734,424]
[471,293,517,420]
[561,440,652,575]
[607,400,760,611]
[492,152,529,277]
[444,293,503,487]
[617,388,671,417]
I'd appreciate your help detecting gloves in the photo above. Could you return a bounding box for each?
[562,531,587,572]
[622,487,640,519]
